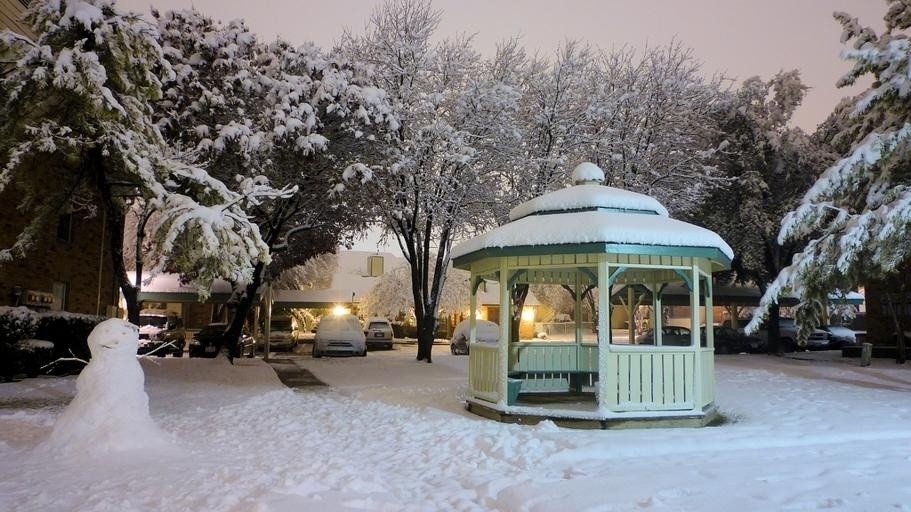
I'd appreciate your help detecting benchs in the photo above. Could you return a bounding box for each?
[507,376,523,403]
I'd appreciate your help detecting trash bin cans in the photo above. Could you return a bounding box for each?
[507,378,523,406]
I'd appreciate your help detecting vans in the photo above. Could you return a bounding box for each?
[257,315,298,352]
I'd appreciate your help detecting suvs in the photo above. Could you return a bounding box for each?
[137,305,184,359]
[360,316,394,350]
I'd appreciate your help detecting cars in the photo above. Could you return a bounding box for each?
[310,314,366,359]
[188,321,260,363]
[448,314,504,358]
[635,309,911,354]
